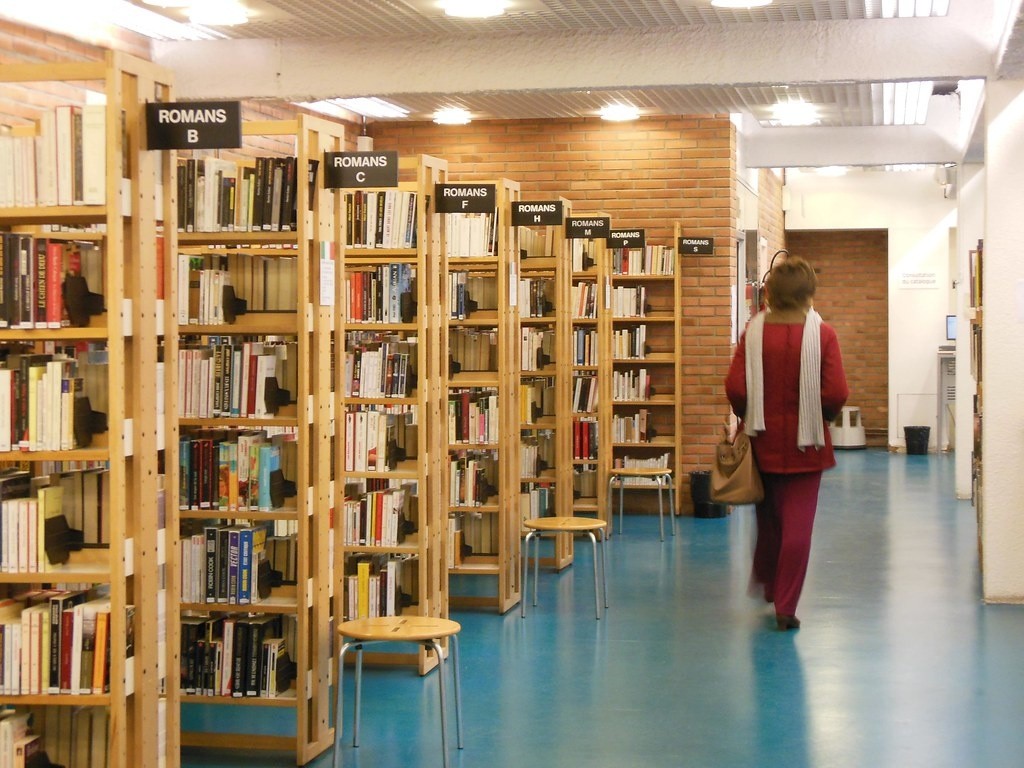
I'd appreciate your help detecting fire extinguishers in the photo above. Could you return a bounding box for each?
[760,281,771,311]
[746,278,756,327]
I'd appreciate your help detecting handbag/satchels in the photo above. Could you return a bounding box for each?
[707,408,767,505]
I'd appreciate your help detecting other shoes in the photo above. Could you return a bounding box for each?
[774,616,802,632]
[762,578,776,603]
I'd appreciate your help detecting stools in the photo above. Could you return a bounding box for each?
[333,615,462,768]
[521,516,609,620]
[606,467,677,543]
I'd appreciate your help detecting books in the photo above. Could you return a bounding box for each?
[1,103,675,767]
[969,238,982,544]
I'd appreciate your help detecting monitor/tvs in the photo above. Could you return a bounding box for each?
[946,315,957,341]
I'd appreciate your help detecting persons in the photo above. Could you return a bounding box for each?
[725,259,849,632]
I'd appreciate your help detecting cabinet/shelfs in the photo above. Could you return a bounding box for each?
[0,48,681,767]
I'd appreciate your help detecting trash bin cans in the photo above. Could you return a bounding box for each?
[903,425,931,455]
